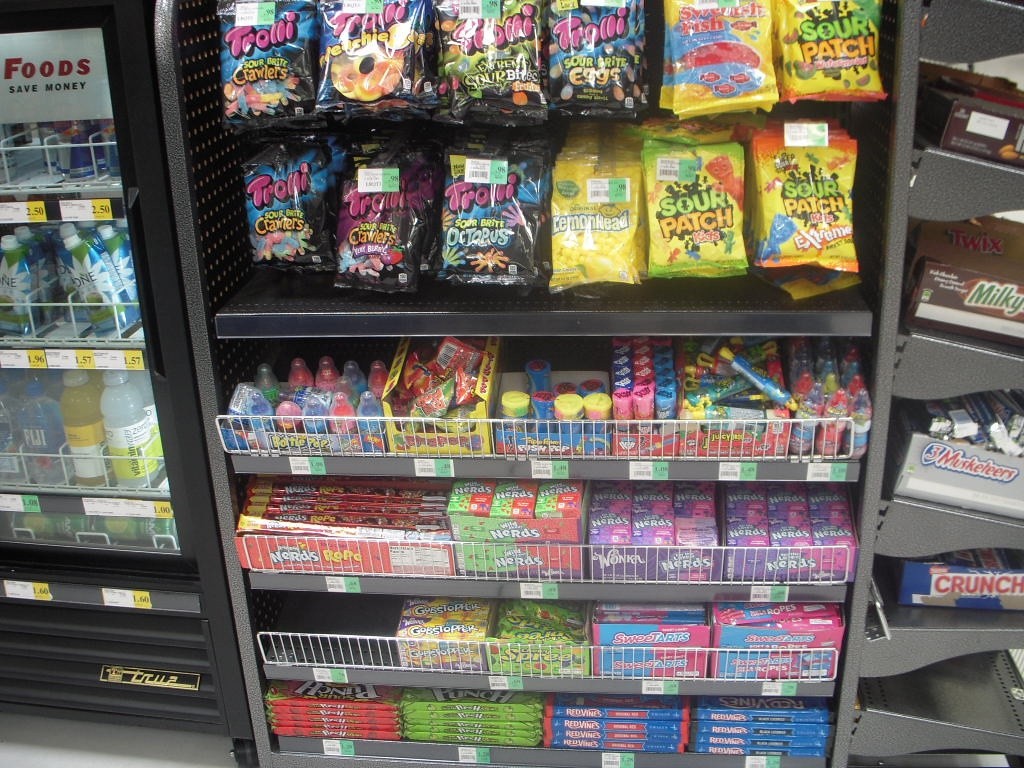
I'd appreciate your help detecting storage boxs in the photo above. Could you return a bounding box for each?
[886,66,1024,612]
[221,371,860,759]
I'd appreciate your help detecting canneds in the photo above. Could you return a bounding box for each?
[0,123,18,183]
[9,511,179,541]
[36,118,123,178]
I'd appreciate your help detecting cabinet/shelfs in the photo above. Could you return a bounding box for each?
[153,0,1024,768]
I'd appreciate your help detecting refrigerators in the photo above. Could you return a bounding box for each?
[0,1,248,735]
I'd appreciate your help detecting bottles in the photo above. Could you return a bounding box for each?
[787,341,871,458]
[252,355,390,435]
[0,119,166,490]
